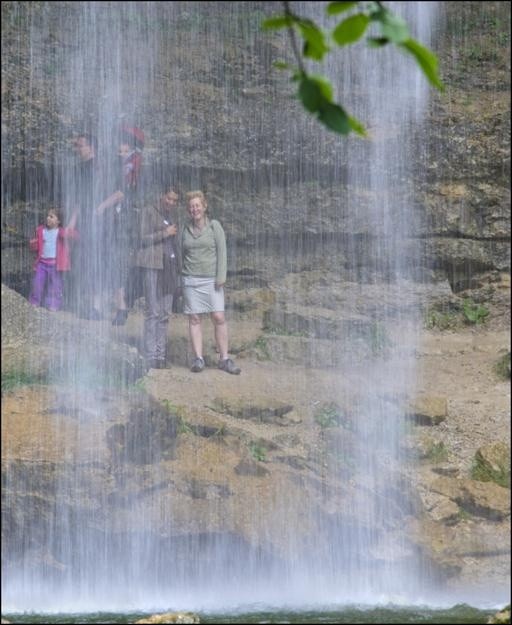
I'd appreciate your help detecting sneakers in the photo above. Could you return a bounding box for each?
[112,310,126,327]
[82,308,100,320]
[151,358,172,369]
[192,358,205,372]
[218,358,241,375]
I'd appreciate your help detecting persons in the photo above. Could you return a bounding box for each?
[174,190,242,375]
[63,133,111,321]
[133,185,182,374]
[95,124,147,328]
[30,205,81,309]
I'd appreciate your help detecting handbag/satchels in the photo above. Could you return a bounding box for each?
[162,251,179,285]
